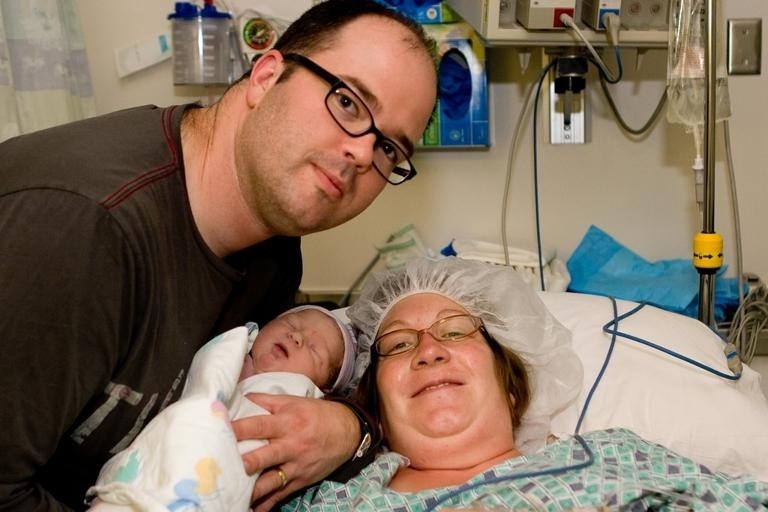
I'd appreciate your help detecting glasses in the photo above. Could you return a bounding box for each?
[251,54,416,185]
[371,315,495,359]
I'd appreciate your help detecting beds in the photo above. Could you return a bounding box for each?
[321,301,765,512]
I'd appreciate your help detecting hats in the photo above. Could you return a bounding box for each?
[276,304,357,394]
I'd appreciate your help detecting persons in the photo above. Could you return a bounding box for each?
[0,1,438,512]
[263,254,767,512]
[235,302,357,395]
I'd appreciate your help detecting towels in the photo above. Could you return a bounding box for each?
[454,230,559,269]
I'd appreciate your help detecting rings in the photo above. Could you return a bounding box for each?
[272,466,288,489]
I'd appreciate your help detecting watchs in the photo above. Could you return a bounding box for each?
[348,404,372,461]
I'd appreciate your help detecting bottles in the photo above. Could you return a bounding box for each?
[168,2,232,85]
